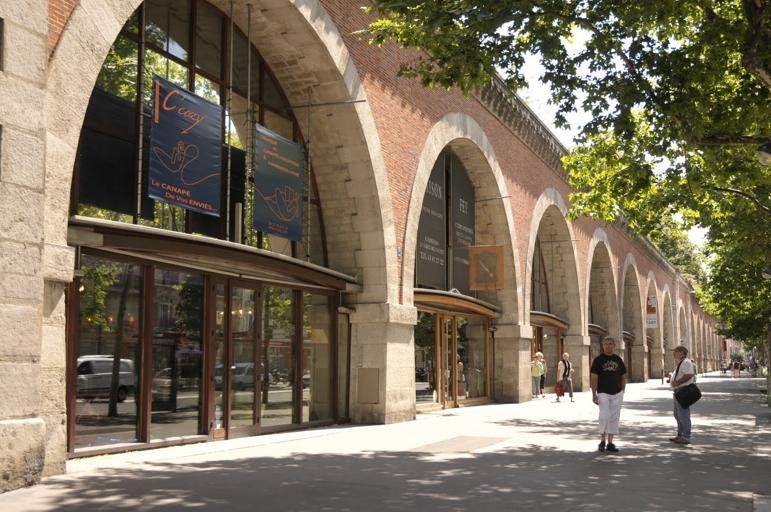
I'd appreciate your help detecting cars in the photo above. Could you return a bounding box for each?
[73,355,254,402]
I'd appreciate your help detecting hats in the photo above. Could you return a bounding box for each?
[670,346,688,355]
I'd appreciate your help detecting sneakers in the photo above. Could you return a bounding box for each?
[599,441,605,455]
[607,444,618,452]
[670,435,690,444]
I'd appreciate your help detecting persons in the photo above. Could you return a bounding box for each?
[531,354,544,398]
[536,351,546,397]
[591,337,626,451]
[457,354,468,399]
[669,346,697,445]
[434,359,443,402]
[556,353,575,402]
[720,358,757,377]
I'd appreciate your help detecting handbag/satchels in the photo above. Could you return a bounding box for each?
[556,384,564,396]
[674,383,701,409]
[458,382,466,396]
